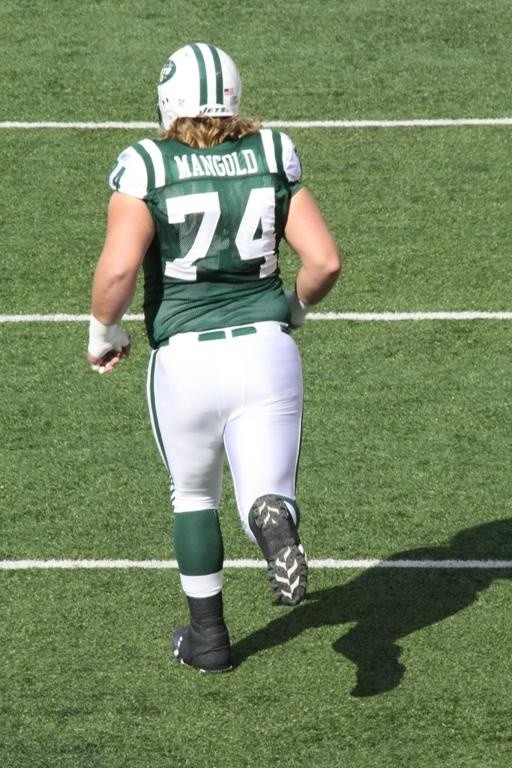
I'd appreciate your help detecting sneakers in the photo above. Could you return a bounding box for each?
[247,494,308,605]
[173,623,231,674]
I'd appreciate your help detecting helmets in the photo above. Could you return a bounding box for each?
[157,42,241,132]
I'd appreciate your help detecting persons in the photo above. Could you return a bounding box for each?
[87,43,342,675]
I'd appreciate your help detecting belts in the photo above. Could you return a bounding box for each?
[154,322,293,349]
